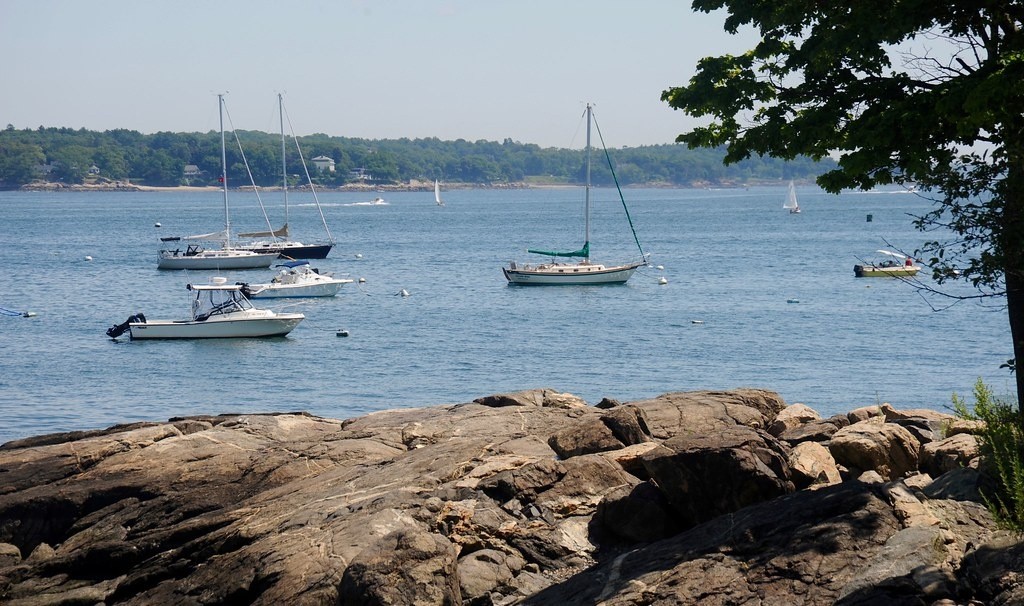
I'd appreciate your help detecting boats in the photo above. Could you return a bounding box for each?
[853,250,921,278]
[235,253,354,298]
[105,277,305,341]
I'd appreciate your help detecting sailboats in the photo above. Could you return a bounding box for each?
[502,101,650,284]
[221,93,338,258]
[782,179,801,214]
[434,178,446,206]
[156,89,281,269]
